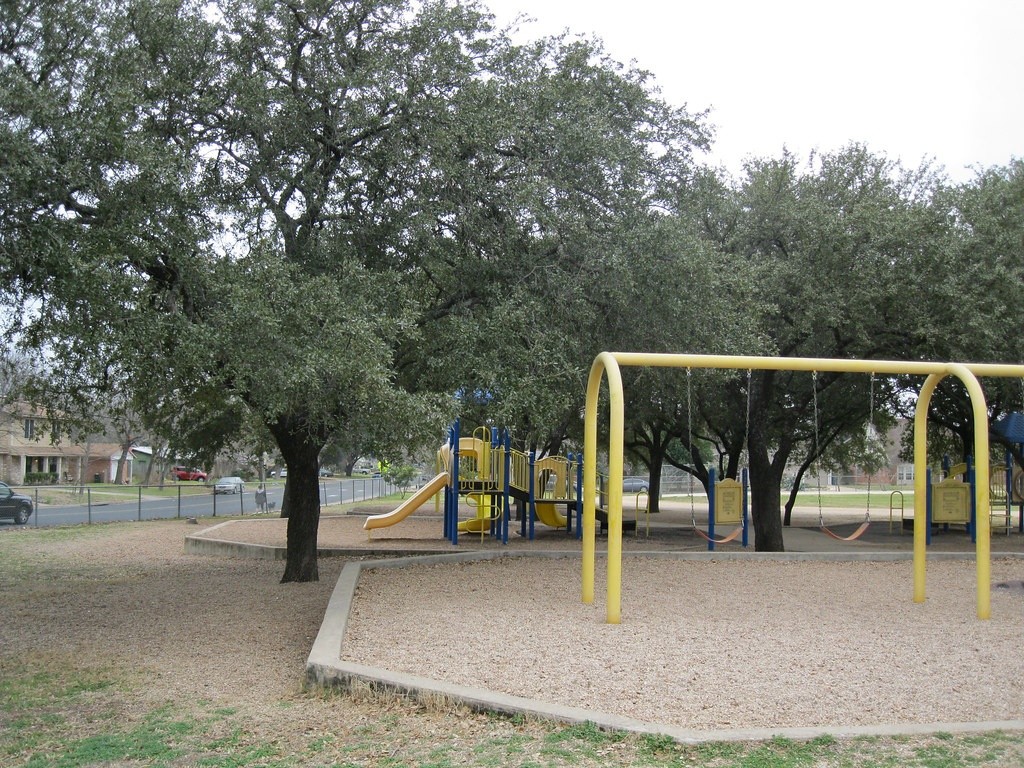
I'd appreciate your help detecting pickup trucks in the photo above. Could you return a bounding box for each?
[353,466,370,475]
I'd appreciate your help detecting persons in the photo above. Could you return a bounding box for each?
[255,486,266,513]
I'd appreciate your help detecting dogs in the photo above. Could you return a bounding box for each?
[268,502,276,513]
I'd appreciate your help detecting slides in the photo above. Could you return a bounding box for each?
[535,504,567,527]
[363,472,449,530]
[458,493,504,531]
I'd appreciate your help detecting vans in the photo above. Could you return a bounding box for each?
[419,474,435,487]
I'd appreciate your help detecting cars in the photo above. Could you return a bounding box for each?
[372,472,382,478]
[0,482,33,524]
[544,475,578,491]
[319,469,333,477]
[214,477,246,494]
[623,478,649,493]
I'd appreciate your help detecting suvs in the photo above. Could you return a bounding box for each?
[271,469,287,478]
[172,466,208,482]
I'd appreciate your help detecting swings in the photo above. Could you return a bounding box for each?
[685,367,753,545]
[812,371,876,542]
